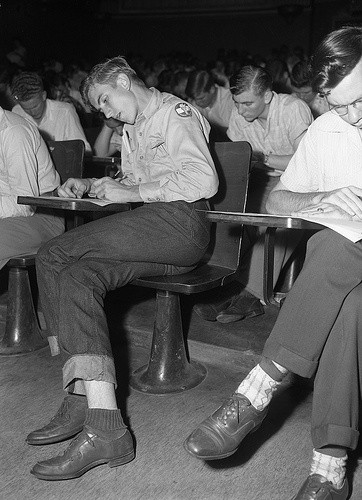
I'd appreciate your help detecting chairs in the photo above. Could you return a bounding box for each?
[0,140,328,396]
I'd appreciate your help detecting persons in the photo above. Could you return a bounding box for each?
[0,42,125,276]
[182,24,362,499]
[129,47,335,323]
[26,57,219,480]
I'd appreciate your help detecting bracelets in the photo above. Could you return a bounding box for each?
[263,155,269,162]
[92,178,98,180]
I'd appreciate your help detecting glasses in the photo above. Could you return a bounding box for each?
[328,99,362,116]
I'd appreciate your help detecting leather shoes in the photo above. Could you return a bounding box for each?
[295,475,349,500]
[31,428,134,480]
[193,296,230,321]
[184,391,268,459]
[217,293,264,324]
[27,395,88,446]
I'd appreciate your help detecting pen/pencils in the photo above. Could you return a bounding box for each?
[112,170,120,179]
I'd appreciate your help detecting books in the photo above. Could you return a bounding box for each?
[292,200,362,243]
